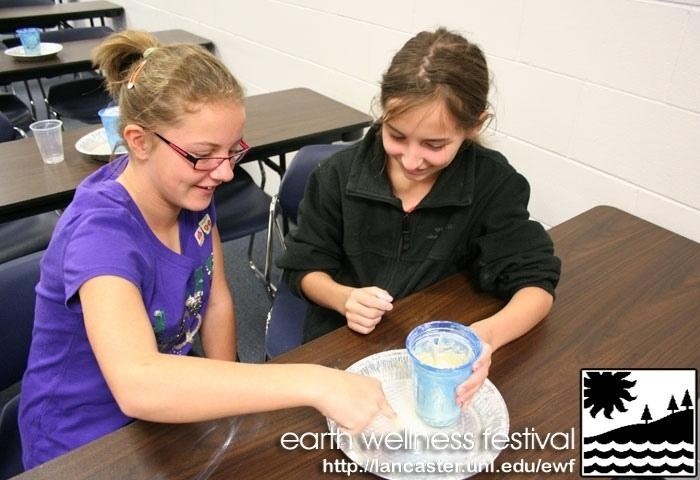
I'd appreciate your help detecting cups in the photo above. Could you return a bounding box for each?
[404,321,479,428]
[99,111,129,146]
[30,117,66,162]
[19,30,44,53]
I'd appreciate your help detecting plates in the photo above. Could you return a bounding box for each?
[4,45,66,57]
[75,127,145,159]
[324,346,509,480]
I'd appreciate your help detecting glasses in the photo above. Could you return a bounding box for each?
[136,124,251,172]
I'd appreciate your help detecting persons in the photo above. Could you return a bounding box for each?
[16,26,400,473]
[273,27,561,406]
